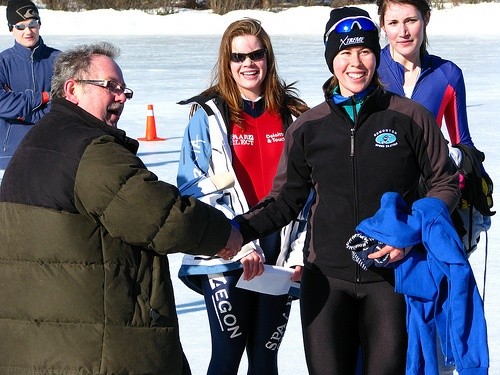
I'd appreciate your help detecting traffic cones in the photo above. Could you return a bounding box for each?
[135,104,167,141]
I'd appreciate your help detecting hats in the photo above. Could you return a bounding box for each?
[324,7,381,71]
[6,0,40,31]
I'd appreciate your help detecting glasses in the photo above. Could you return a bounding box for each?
[9,19,40,30]
[478,175,493,208]
[325,15,381,37]
[75,77,133,99]
[230,48,266,62]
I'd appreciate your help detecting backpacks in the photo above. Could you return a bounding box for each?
[449,144,496,254]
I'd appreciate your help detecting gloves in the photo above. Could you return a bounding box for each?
[346,232,389,272]
[2,83,24,120]
